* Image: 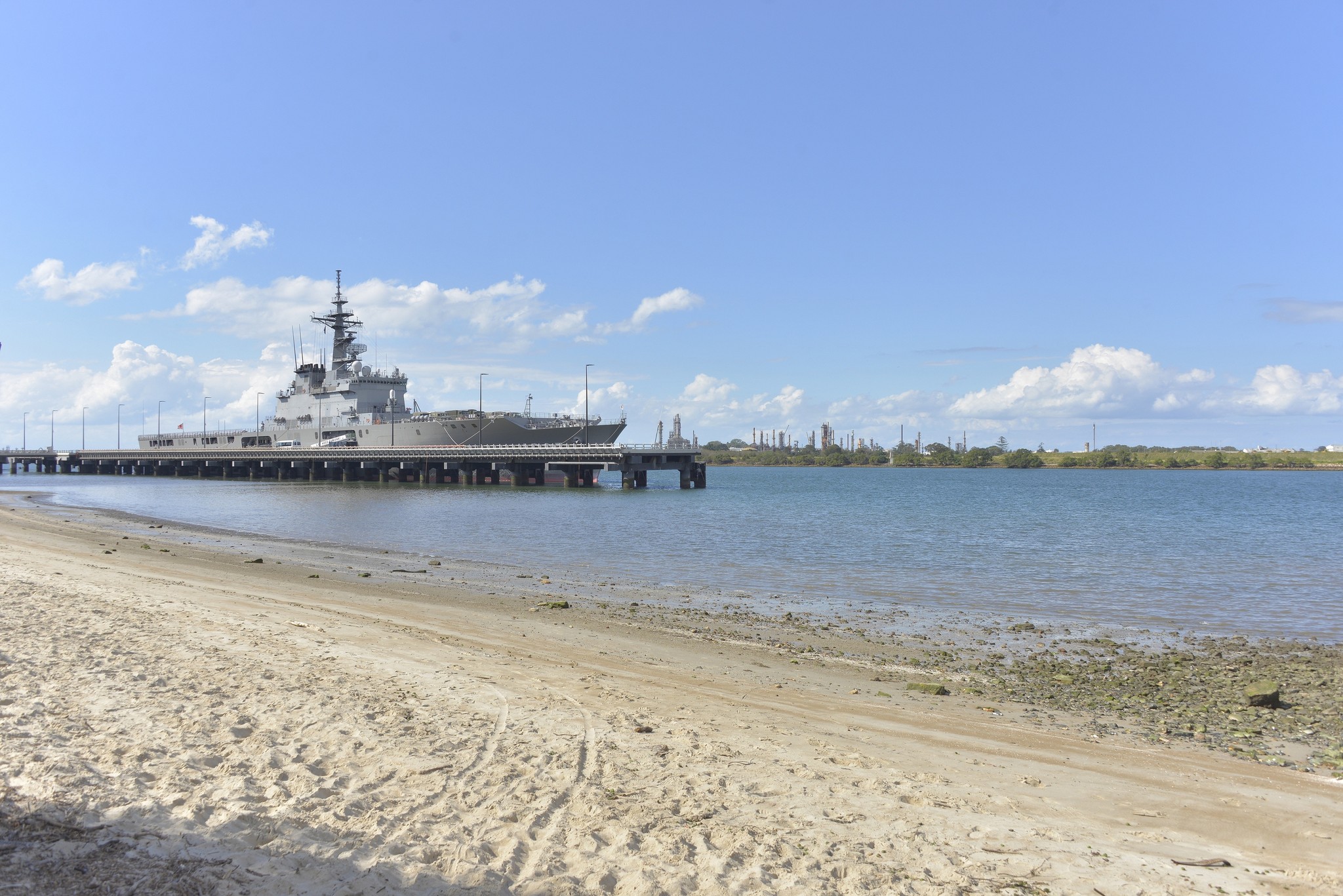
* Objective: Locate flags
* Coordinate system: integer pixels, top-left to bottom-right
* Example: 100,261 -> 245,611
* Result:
324,326 -> 326,334
178,423 -> 183,429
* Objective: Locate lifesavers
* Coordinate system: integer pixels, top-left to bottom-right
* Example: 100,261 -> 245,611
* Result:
476,414 -> 480,418
366,419 -> 369,422
621,419 -> 625,423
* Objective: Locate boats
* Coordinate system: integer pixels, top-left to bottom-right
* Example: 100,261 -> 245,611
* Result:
728,446 -> 743,452
138,268 -> 629,470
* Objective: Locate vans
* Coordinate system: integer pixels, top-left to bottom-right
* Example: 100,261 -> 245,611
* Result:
274,440 -> 301,448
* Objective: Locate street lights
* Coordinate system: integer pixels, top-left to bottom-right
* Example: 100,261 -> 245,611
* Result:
82,407 -> 89,450
391,381 -> 399,449
52,410 -> 58,446
157,401 -> 166,449
585,364 -> 595,448
1093,424 -> 1096,450
256,392 -> 264,448
118,404 -> 125,450
479,373 -> 489,448
204,396 -> 212,449
320,385 -> 328,447
24,412 -> 30,450
